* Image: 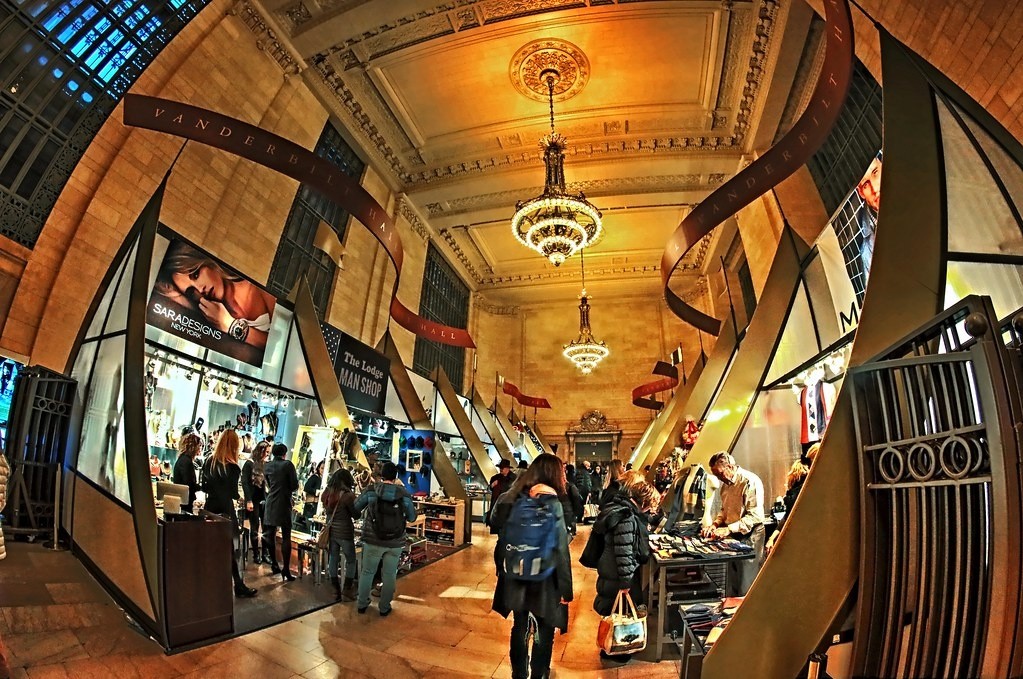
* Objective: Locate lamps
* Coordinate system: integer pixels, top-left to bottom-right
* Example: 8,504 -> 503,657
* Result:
562,248 -> 609,378
148,348 -> 290,407
510,78 -> 603,269
354,413 -> 399,433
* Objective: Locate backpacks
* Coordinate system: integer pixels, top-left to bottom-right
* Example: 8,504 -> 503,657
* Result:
368,490 -> 406,540
505,492 -> 560,581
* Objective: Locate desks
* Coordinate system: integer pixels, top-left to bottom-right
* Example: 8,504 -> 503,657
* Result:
649,537 -> 755,663
679,597 -> 739,679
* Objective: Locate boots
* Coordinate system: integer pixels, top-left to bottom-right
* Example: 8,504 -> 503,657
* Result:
261,537 -> 271,564
250,533 -> 263,563
233,579 -> 258,597
333,584 -> 341,600
342,577 -> 356,600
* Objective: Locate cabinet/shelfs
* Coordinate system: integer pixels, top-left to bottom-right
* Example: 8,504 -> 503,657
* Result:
406,498 -> 464,547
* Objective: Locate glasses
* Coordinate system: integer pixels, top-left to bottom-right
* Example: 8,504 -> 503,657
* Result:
621,465 -> 625,468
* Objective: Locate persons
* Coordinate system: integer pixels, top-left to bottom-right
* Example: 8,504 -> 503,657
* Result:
241,441 -> 273,565
783,442 -> 821,520
354,467 -> 375,495
174,433 -> 202,515
263,442 -> 299,582
488,460 -> 652,534
490,453 -> 573,679
673,462 -> 720,522
0,433 -> 11,679
202,428 -> 259,597
153,239 -> 276,348
354,461 -> 418,616
593,481 -> 661,664
303,461 -> 325,530
856,150 -> 881,288
321,469 -> 362,602
702,451 -> 766,597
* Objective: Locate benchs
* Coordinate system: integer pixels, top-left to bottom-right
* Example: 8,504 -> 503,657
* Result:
244,520 -> 316,579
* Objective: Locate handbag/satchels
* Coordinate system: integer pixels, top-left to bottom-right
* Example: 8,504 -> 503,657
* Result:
596,590 -> 647,655
582,493 -> 600,521
579,529 -> 604,568
316,526 -> 329,549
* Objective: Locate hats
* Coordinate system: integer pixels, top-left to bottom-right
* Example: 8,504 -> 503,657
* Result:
396,436 -> 436,487
381,462 -> 396,479
496,459 -> 513,469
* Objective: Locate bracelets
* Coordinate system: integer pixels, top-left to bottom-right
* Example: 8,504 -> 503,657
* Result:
228,318 -> 249,343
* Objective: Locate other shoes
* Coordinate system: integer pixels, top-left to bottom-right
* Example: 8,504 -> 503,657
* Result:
380,607 -> 391,617
357,606 -> 367,614
600,649 -> 631,663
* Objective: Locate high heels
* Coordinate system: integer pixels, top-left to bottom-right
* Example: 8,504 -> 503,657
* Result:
282,569 -> 295,581
271,562 -> 282,574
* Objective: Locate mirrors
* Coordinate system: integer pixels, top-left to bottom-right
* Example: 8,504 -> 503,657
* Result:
278,425 -> 333,540
